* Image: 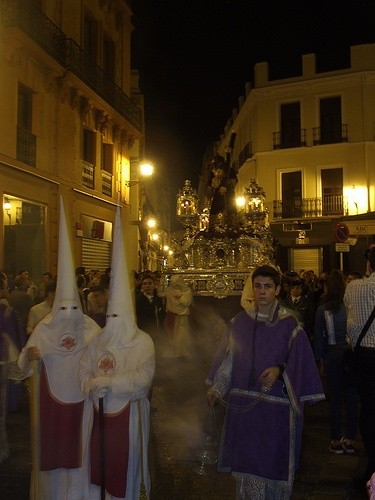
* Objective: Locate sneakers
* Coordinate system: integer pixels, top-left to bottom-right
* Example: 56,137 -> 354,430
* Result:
329,437 -> 354,454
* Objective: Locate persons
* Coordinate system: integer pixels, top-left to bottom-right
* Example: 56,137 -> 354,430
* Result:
18,195 -> 102,500
78,207 -> 156,500
0,269 -> 198,464
205,264 -> 327,500
276,246 -> 375,500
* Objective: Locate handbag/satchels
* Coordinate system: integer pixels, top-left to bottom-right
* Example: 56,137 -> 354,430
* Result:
340,349 -> 360,387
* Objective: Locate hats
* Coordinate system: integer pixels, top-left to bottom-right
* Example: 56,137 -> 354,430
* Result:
288,280 -> 305,287
209,155 -> 226,168
